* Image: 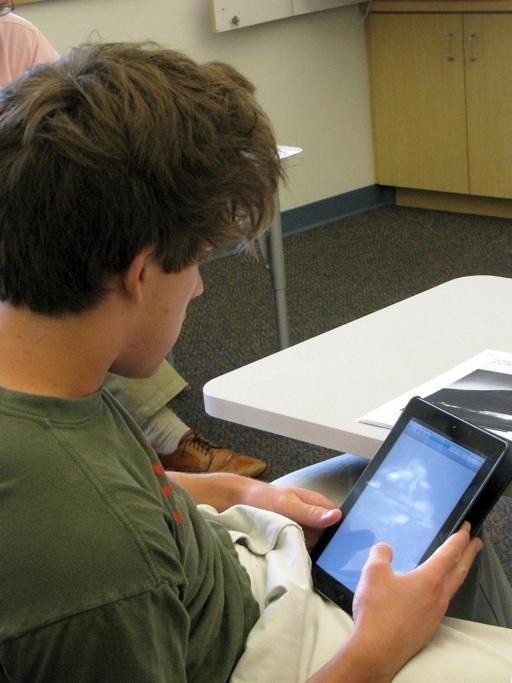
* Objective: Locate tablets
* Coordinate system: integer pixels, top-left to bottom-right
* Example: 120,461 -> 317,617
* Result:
311,396 -> 509,620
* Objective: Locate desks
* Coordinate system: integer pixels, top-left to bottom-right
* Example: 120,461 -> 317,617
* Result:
202,274 -> 512,461
266,143 -> 306,349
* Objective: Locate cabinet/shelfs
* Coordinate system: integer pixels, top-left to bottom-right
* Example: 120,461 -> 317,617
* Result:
364,12 -> 512,221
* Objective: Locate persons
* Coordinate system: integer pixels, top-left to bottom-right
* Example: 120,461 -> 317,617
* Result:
0,37 -> 512,683
0,1 -> 268,479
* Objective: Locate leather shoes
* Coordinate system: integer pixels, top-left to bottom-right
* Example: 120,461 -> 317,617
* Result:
158,429 -> 267,479
